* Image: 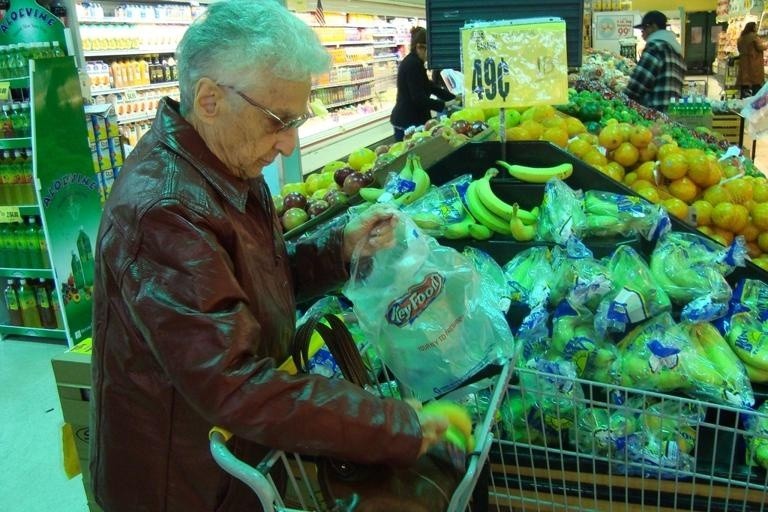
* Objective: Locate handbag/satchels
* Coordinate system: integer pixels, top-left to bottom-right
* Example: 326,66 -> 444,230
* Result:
292,311 -> 490,511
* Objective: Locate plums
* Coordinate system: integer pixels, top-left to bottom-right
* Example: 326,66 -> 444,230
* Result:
275,118 -> 488,234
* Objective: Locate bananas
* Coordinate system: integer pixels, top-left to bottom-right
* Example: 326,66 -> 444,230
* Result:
346,153 -> 768,466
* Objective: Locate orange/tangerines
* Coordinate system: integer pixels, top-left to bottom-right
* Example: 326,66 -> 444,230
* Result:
558,81 -> 766,177
488,104 -> 767,271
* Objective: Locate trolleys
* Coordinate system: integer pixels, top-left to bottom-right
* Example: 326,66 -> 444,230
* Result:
207,292 -> 767,511
715,55 -> 741,100
682,65 -> 710,98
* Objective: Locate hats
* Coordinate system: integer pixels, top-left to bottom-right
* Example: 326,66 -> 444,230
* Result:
632,11 -> 668,29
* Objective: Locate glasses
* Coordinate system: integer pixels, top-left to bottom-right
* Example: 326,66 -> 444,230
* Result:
214,82 -> 313,133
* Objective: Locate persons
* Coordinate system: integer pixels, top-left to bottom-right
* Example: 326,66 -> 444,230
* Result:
89,0 -> 448,512
737,21 -> 768,100
626,11 -> 686,114
390,28 -> 457,142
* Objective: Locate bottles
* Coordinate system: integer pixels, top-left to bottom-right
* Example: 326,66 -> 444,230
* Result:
77,3 -> 406,151
1,42 -> 67,331
70,250 -> 85,289
621,45 -> 638,62
666,91 -> 714,131
77,223 -> 97,287
